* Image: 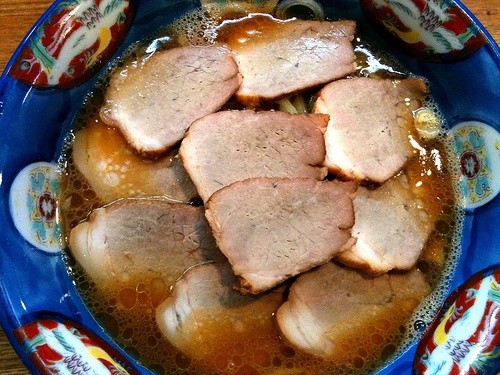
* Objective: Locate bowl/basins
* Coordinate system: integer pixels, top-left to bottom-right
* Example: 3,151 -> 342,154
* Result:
0,0 -> 500,375
